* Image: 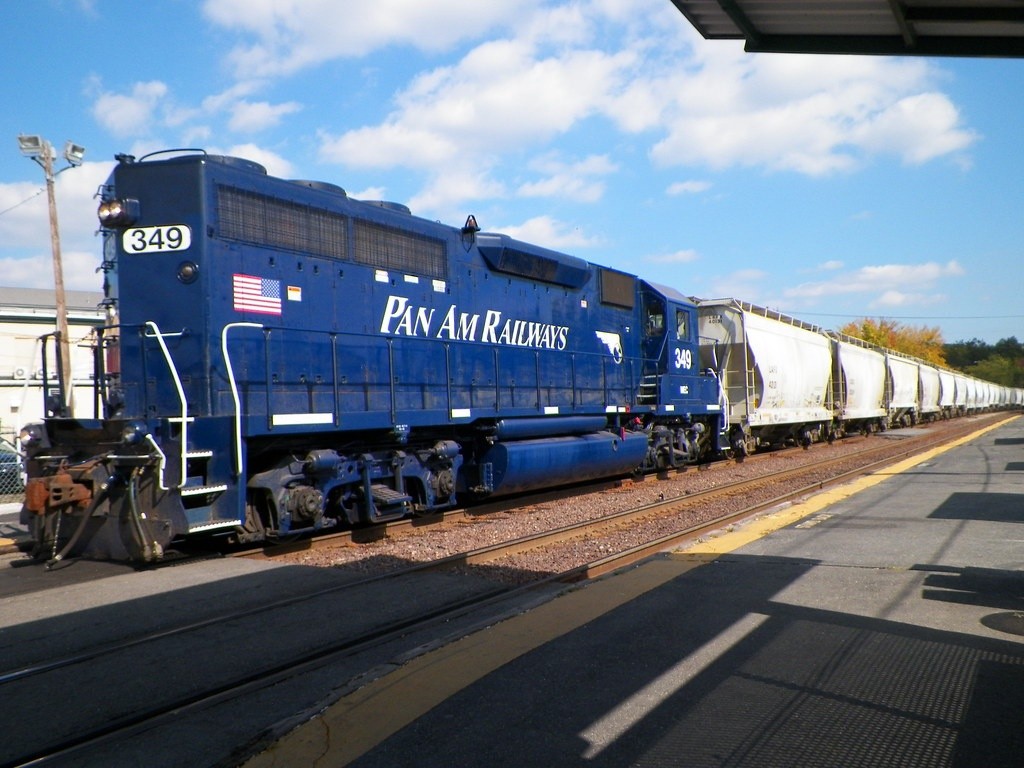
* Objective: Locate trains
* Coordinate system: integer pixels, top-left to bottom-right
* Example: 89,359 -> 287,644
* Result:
15,142 -> 1024,572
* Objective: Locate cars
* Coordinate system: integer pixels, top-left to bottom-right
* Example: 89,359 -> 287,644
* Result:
0,438 -> 27,495
